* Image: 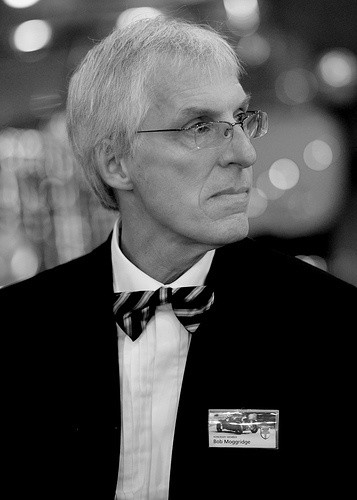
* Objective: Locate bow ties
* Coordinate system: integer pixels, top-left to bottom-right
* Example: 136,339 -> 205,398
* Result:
109,288 -> 214,342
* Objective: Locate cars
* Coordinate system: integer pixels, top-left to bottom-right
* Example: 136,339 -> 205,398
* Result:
216,416 -> 258,434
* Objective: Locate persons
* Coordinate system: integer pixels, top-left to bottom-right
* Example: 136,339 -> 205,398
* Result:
0,18 -> 357,500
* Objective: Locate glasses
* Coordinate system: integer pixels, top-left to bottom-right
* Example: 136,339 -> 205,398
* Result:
134,110 -> 269,147
